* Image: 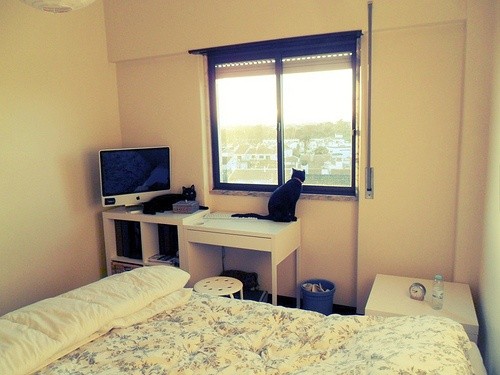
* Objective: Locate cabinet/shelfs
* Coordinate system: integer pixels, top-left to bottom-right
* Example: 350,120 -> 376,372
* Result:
101,206 -> 224,288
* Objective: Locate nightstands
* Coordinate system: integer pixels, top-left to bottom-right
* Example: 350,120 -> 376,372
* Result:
364,273 -> 479,345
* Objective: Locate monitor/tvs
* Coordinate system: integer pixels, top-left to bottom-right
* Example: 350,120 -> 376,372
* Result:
98,146 -> 172,214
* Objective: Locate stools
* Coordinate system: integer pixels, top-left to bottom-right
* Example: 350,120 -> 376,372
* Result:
194,276 -> 243,301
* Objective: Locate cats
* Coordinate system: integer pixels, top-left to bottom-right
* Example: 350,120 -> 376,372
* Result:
232,168 -> 306,222
143,184 -> 209,216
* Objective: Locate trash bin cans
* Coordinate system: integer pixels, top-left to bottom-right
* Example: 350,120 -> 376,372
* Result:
299,277 -> 337,316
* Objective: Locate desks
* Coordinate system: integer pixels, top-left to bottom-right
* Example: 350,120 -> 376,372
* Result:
186,212 -> 302,310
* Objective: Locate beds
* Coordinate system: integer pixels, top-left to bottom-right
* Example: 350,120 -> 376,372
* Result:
0,265 -> 491,375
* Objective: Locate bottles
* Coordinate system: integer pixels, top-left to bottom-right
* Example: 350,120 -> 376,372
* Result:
430,272 -> 444,310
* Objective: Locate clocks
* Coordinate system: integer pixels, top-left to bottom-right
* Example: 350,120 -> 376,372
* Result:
409,283 -> 426,301
